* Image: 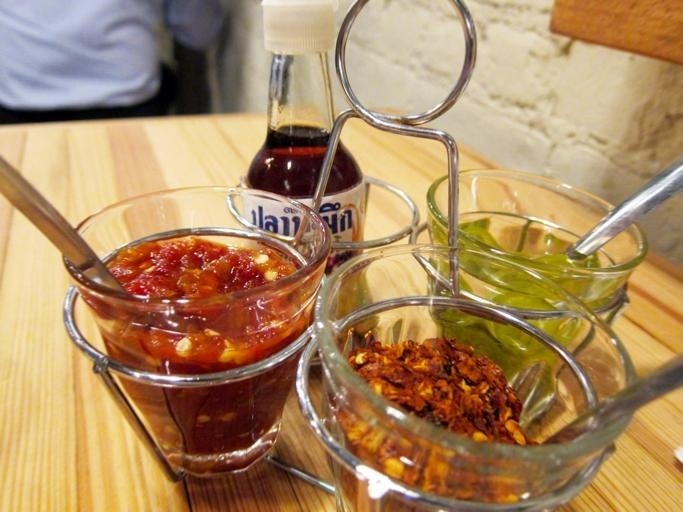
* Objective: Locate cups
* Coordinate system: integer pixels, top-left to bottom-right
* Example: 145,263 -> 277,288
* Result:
63,187 -> 327,480
427,166 -> 647,427
314,243 -> 640,512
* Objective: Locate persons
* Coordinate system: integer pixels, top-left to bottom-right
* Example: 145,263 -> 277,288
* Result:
0,1 -> 225,125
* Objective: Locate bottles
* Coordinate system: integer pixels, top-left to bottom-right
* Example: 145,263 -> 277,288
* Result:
244,0 -> 371,382
